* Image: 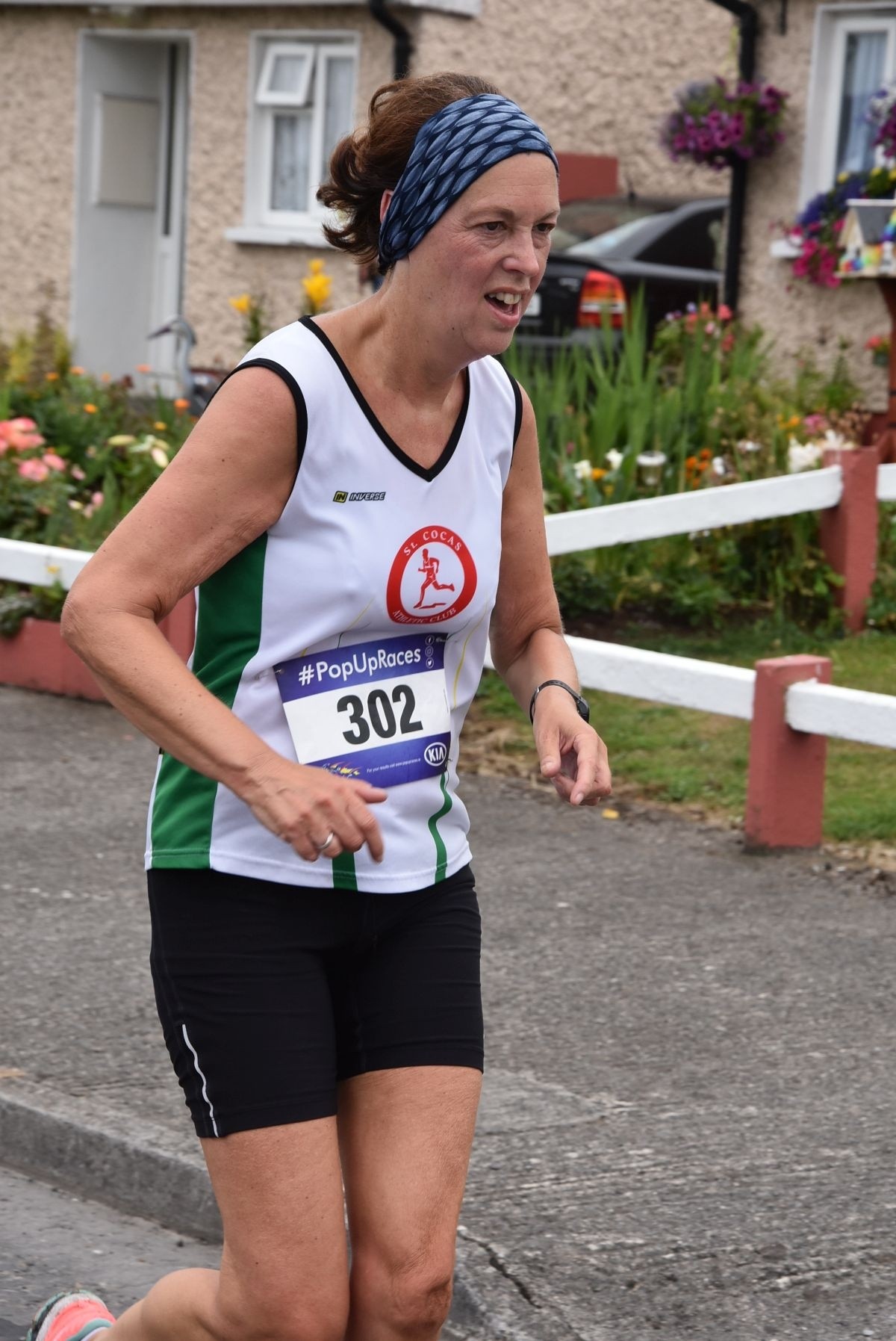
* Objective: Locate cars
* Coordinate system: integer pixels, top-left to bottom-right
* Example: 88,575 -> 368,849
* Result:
506,187 -> 727,369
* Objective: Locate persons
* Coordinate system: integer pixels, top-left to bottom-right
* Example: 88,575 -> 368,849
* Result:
21,70 -> 612,1341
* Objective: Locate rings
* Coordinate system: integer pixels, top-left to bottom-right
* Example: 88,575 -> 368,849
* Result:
315,831 -> 334,849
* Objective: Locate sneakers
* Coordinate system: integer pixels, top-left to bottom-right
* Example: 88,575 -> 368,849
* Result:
28,1289 -> 119,1341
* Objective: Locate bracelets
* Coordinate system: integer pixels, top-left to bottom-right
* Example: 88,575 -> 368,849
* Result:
529,680 -> 590,724
